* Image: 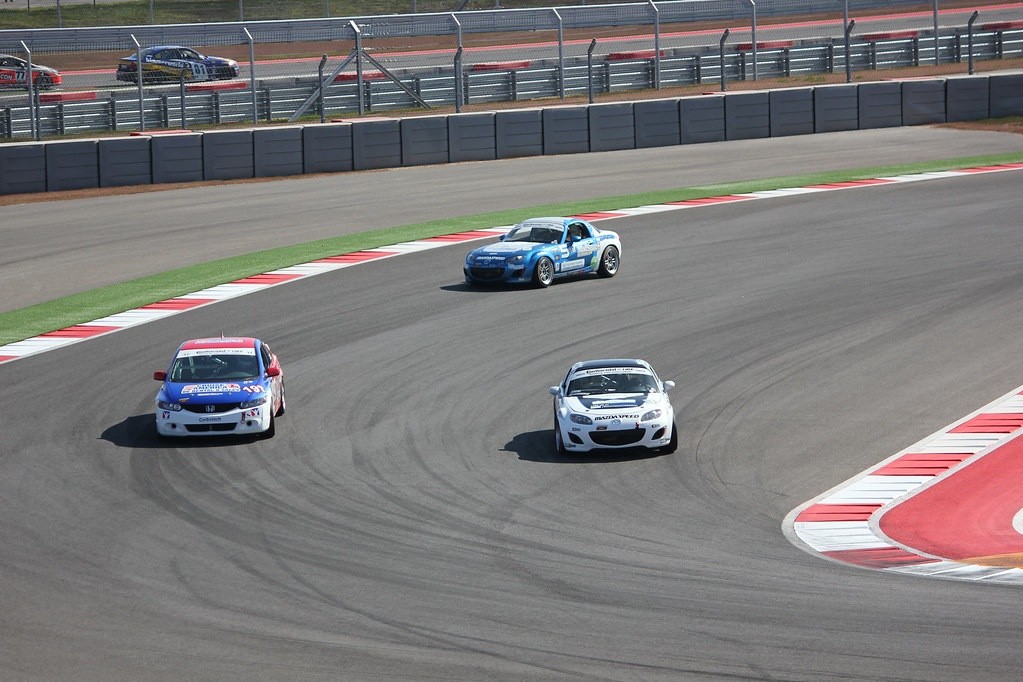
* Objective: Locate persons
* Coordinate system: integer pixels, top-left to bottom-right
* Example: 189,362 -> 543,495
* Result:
215,358 -> 241,376
615,378 -> 651,391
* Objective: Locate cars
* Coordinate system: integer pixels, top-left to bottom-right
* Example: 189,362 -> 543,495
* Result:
154,332 -> 287,440
0,51 -> 63,90
547,354 -> 678,456
463,215 -> 624,286
117,45 -> 242,84
